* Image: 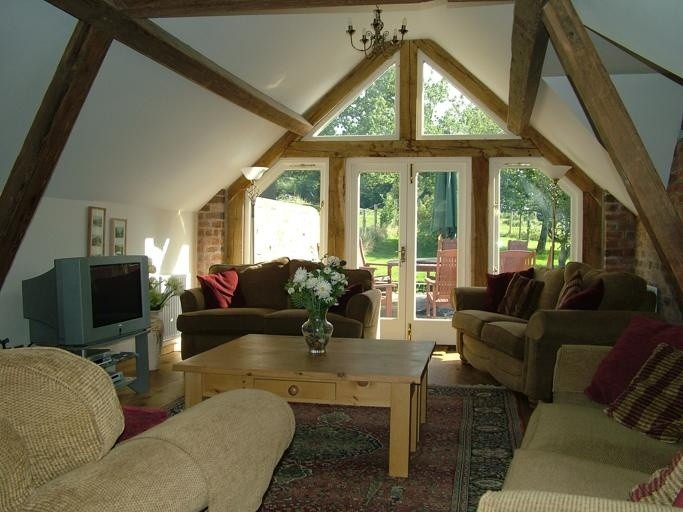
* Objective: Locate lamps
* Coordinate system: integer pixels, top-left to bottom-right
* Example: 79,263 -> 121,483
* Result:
241,166 -> 269,264
344,2 -> 409,59
540,164 -> 572,267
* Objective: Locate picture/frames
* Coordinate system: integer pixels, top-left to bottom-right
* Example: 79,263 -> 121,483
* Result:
85,206 -> 128,257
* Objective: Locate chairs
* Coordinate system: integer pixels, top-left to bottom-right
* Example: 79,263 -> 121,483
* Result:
0,346 -> 296,509
424,232 -> 535,318
356,237 -> 397,316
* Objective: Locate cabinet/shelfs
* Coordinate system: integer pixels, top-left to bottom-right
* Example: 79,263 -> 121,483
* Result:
70,329 -> 150,396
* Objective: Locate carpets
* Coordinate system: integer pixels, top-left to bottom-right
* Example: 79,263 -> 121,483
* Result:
157,383 -> 529,512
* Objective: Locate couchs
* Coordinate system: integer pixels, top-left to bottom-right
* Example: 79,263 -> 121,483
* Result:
174,254 -> 381,387
452,260 -> 664,409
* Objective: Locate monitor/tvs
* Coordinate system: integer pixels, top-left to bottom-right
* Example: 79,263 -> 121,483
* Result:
21,255 -> 150,345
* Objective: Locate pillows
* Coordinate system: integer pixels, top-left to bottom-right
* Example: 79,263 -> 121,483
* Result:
555,269 -> 605,310
585,316 -> 681,441
482,265 -> 544,318
194,268 -> 245,311
626,447 -> 683,510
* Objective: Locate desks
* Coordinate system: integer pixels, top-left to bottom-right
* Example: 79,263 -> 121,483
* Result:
387,256 -> 441,284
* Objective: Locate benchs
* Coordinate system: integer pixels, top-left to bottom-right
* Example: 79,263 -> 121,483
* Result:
480,342 -> 682,510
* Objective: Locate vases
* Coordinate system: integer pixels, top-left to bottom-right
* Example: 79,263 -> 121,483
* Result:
300,310 -> 335,355
147,311 -> 165,371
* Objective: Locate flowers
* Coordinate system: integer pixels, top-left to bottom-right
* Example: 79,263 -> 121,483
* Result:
147,263 -> 183,311
282,255 -> 350,344
